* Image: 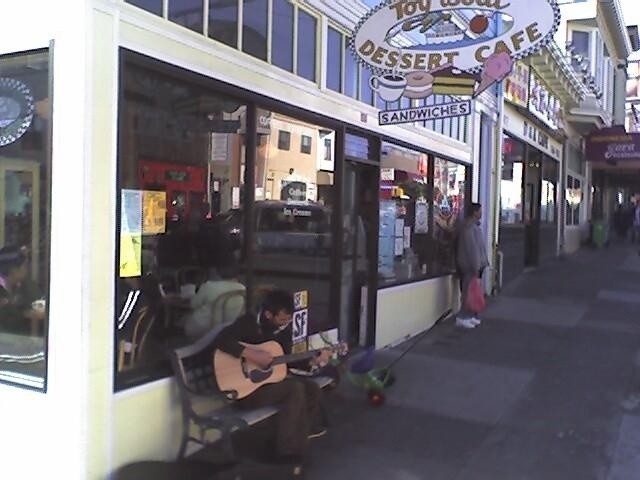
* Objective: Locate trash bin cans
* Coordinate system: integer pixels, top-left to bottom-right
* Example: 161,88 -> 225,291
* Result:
591,222 -> 611,248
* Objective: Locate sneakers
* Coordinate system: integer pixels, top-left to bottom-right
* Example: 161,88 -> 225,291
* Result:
455,315 -> 481,330
306,429 -> 328,440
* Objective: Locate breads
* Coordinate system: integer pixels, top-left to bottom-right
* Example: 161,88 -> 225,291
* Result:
402,72 -> 434,99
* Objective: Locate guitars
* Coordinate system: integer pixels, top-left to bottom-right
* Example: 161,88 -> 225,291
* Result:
214,341 -> 348,402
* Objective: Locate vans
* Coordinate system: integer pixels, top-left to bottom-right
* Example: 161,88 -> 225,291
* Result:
252,199 -> 334,258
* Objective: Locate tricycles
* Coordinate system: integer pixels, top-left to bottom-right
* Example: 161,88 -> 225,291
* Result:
313,308 -> 455,404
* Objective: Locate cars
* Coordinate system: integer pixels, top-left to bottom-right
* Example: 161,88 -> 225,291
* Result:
217,207 -> 241,250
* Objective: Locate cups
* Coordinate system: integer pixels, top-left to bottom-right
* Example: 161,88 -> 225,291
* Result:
367,73 -> 407,103
32,300 -> 46,312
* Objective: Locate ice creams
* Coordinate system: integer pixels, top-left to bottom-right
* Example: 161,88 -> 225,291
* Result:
472,52 -> 513,98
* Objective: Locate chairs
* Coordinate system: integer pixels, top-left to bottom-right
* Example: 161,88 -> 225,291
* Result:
1,260 -> 254,370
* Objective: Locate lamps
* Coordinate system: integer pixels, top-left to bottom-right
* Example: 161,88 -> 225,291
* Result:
558,38 -> 604,101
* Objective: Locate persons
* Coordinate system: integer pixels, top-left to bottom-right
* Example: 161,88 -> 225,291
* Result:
0,246 -> 247,366
454,203 -> 490,330
629,192 -> 640,245
205,291 -> 327,468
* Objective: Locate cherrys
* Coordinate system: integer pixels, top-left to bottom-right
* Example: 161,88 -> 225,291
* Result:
470,15 -> 487,33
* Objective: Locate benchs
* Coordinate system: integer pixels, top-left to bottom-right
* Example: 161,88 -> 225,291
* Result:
170,319 -> 335,464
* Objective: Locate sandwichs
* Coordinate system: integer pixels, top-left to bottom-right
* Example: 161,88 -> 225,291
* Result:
432,65 -> 475,95
